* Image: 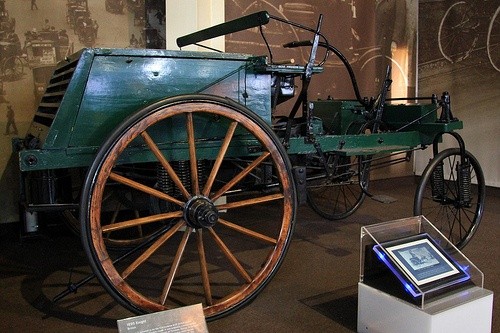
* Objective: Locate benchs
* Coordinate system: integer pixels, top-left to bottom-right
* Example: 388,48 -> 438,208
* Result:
176,11 -> 329,137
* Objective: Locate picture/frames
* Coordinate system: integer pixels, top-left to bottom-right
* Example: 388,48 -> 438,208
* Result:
374,232 -> 470,297
